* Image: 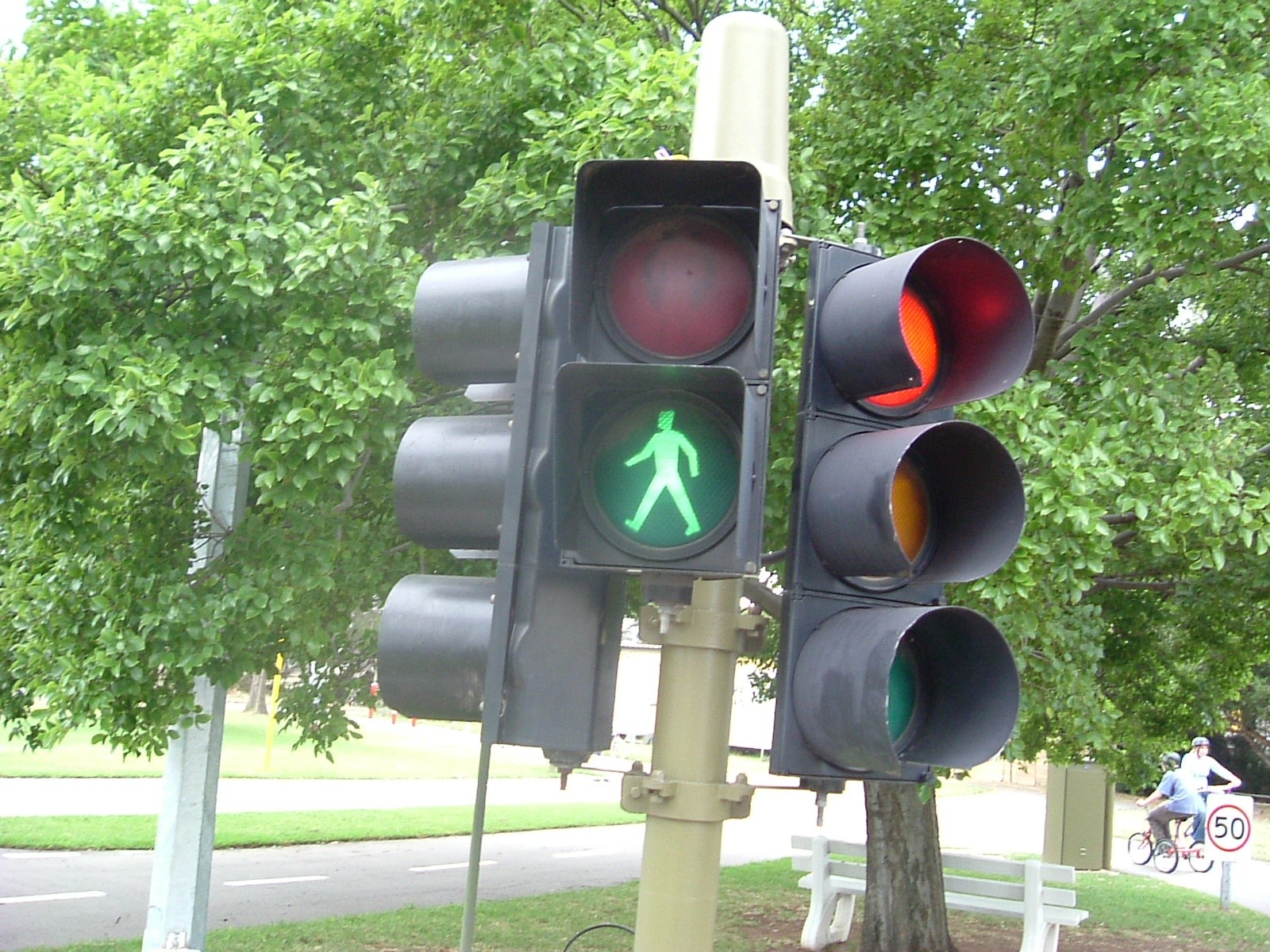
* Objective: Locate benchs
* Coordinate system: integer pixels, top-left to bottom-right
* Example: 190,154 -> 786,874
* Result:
792,836 -> 1089,952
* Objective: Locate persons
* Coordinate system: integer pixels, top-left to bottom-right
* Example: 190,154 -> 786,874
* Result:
1181,737 -> 1241,851
1135,752 -> 1198,856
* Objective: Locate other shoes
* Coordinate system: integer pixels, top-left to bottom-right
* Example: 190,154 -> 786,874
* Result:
1154,844 -> 1168,856
1164,848 -> 1174,858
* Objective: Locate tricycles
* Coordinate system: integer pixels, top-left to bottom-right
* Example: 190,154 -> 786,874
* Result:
1128,805 -> 1214,874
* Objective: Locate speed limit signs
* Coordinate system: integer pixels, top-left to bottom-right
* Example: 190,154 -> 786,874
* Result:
1206,802 -> 1251,852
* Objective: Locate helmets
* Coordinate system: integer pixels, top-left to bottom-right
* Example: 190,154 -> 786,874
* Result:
1159,752 -> 1181,768
1191,736 -> 1209,747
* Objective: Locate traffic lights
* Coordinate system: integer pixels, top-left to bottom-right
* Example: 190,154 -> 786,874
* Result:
769,233 -> 1036,783
547,158 -> 784,578
377,221 -> 626,790
